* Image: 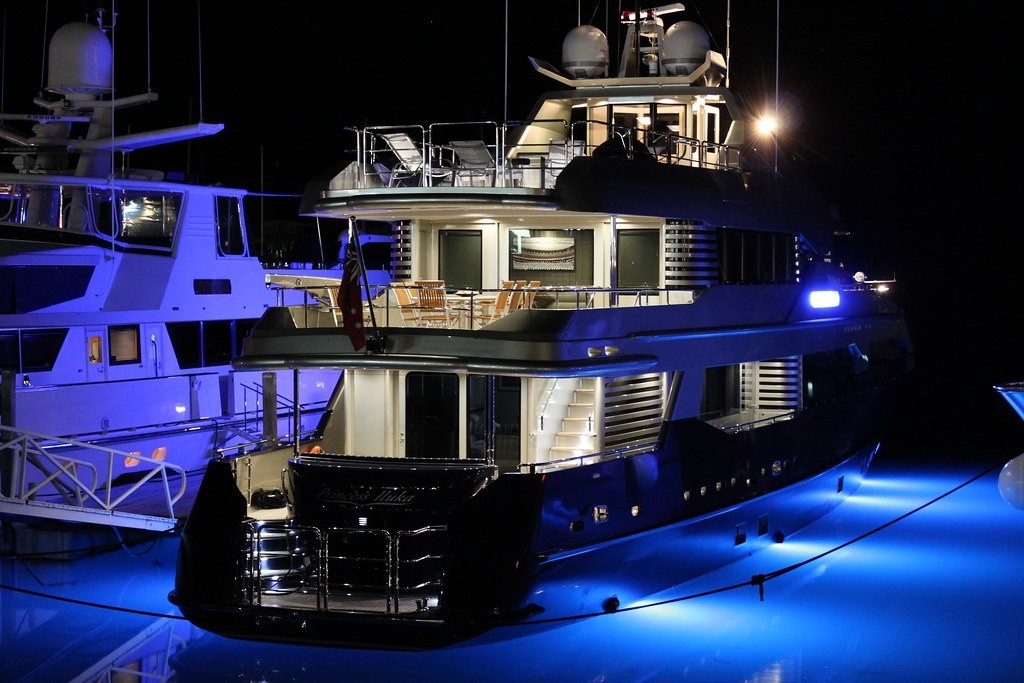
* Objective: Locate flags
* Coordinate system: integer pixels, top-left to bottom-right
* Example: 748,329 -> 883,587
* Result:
336,218 -> 367,352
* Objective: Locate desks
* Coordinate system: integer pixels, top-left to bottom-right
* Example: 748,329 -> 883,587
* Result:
410,295 -> 497,330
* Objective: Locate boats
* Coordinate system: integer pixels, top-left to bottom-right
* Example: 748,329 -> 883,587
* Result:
0,0 -> 394,565
165,0 -> 915,653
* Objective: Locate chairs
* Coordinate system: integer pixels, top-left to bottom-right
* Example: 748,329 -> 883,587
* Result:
379,134 -> 586,192
390,279 -> 541,331
